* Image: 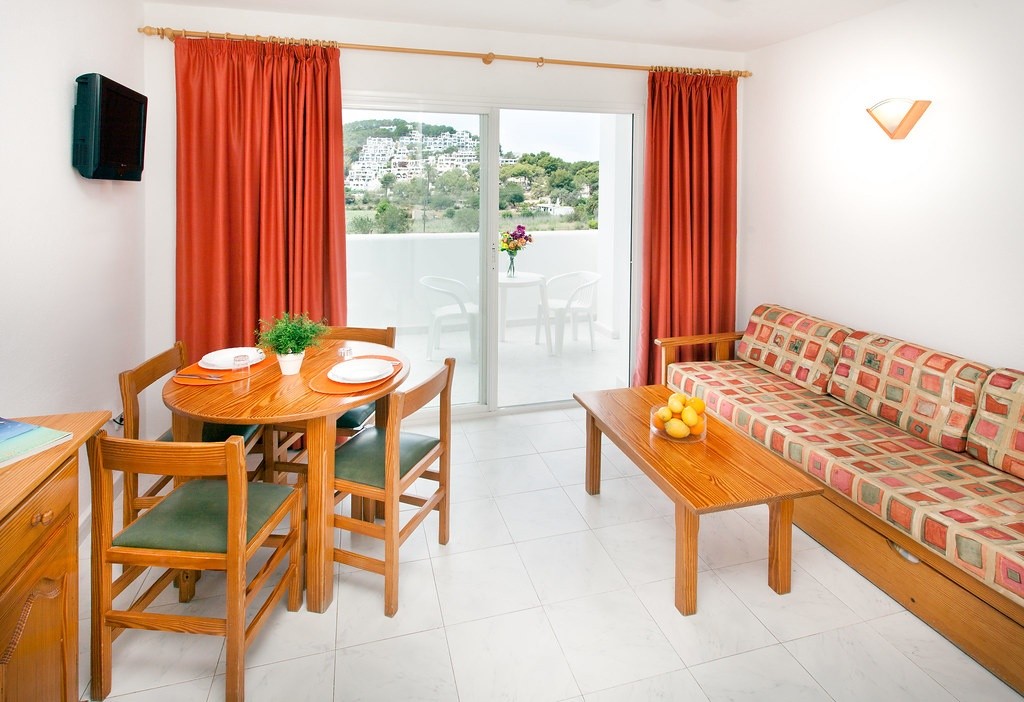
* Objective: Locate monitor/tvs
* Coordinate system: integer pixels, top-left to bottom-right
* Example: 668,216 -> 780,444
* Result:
72,72 -> 148,181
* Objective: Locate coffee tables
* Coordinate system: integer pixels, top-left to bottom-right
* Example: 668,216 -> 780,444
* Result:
573,384 -> 824,615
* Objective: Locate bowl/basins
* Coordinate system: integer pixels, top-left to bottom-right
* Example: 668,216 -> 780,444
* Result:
649,403 -> 708,443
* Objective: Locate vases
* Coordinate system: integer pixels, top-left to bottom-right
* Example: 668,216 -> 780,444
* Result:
505,252 -> 520,277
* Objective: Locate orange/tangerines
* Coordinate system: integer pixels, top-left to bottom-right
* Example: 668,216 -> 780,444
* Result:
652,393 -> 705,435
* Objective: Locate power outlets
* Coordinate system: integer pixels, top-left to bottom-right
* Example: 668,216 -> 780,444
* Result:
113,410 -> 123,430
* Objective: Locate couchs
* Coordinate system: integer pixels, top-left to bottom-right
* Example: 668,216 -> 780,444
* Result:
654,303 -> 1023,701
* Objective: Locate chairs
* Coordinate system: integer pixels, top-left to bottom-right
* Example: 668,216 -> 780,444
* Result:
301,355 -> 455,616
419,275 -> 479,368
272,325 -> 401,524
536,271 -> 602,355
117,337 -> 276,584
78,431 -> 305,702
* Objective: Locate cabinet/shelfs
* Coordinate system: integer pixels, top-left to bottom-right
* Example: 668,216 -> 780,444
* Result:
0,408 -> 117,702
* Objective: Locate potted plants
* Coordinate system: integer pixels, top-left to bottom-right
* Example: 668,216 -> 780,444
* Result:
251,311 -> 328,379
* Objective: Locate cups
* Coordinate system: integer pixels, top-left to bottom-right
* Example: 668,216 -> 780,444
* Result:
231,355 -> 250,394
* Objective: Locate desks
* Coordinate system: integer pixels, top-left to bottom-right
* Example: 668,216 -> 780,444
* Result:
477,270 -> 550,347
162,341 -> 407,613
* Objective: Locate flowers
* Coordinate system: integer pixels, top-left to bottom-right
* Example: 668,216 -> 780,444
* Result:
498,224 -> 535,254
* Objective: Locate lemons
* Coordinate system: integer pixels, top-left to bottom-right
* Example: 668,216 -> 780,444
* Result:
658,399 -> 698,439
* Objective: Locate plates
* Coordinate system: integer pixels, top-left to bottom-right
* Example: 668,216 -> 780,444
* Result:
198,346 -> 266,370
327,359 -> 394,384
